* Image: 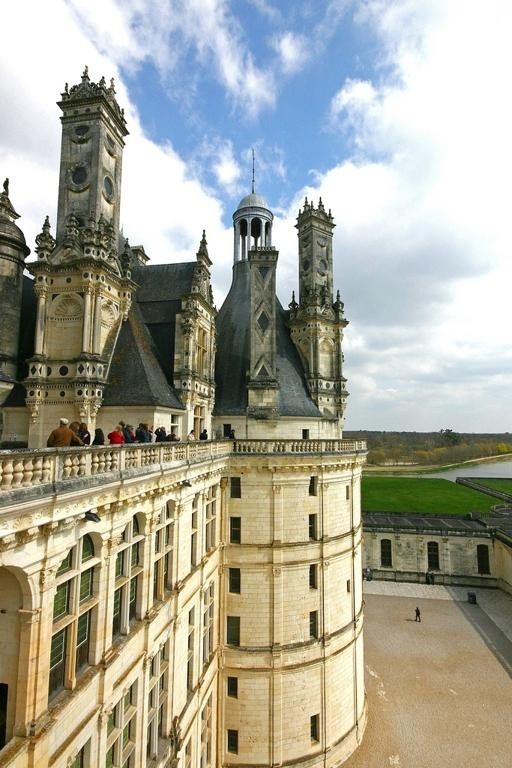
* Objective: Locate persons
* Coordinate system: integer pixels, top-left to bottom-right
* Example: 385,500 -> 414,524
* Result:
365,566 -> 372,581
431,573 -> 435,585
46,418 -> 237,447
426,572 -> 430,585
414,607 -> 421,622
